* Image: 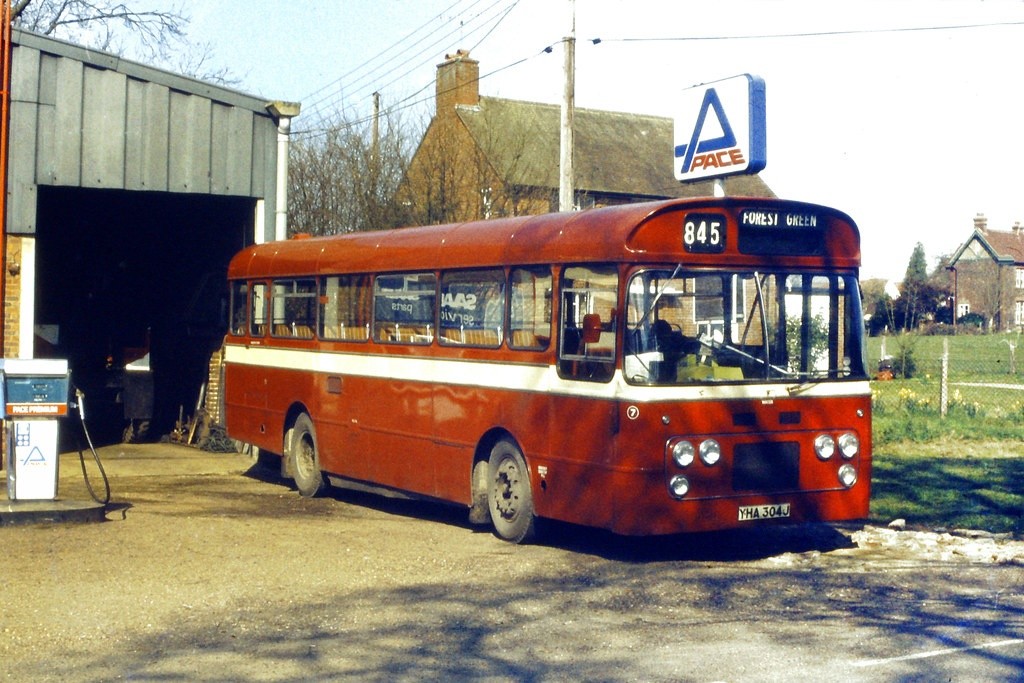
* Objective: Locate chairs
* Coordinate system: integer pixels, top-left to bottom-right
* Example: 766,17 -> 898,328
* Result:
237,321 -> 542,348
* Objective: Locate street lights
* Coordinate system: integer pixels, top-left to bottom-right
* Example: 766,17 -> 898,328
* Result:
944,266 -> 957,334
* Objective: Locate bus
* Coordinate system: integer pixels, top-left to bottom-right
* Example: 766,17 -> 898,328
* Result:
223,194 -> 873,550
